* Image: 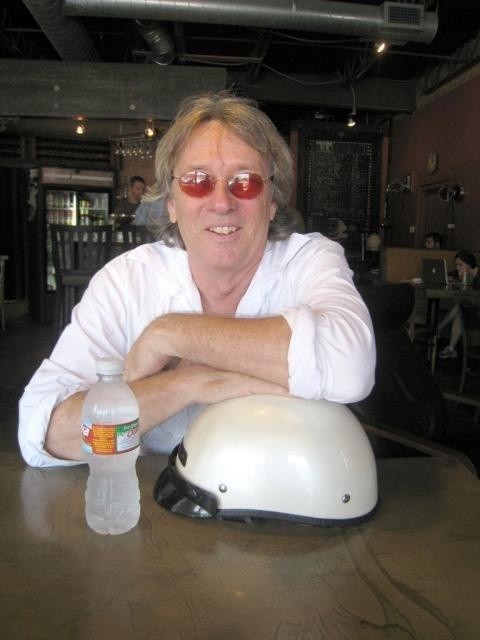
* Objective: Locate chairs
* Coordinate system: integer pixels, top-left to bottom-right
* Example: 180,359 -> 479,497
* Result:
402,283 -> 480,406
46,223 -> 157,341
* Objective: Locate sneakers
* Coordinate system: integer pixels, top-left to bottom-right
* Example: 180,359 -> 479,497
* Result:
437,347 -> 458,358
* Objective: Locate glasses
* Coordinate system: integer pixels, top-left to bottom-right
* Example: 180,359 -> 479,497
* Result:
170,167 -> 275,201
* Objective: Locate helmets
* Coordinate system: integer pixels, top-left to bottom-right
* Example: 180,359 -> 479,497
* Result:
153,393 -> 380,529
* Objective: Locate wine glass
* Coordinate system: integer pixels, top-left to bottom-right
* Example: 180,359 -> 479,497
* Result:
115,143 -> 156,162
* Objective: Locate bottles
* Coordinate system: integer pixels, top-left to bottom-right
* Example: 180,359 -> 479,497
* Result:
80,357 -> 141,537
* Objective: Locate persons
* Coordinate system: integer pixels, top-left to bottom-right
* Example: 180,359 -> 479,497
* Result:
15,98 -> 377,469
115,176 -> 151,230
436,250 -> 480,359
424,232 -> 443,249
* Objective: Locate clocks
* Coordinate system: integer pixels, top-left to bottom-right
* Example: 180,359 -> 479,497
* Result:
426,150 -> 439,174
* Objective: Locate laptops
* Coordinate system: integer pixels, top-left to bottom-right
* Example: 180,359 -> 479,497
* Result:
422,256 -> 456,286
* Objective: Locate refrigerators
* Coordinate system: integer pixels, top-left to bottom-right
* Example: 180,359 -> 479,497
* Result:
40,167 -> 116,294
